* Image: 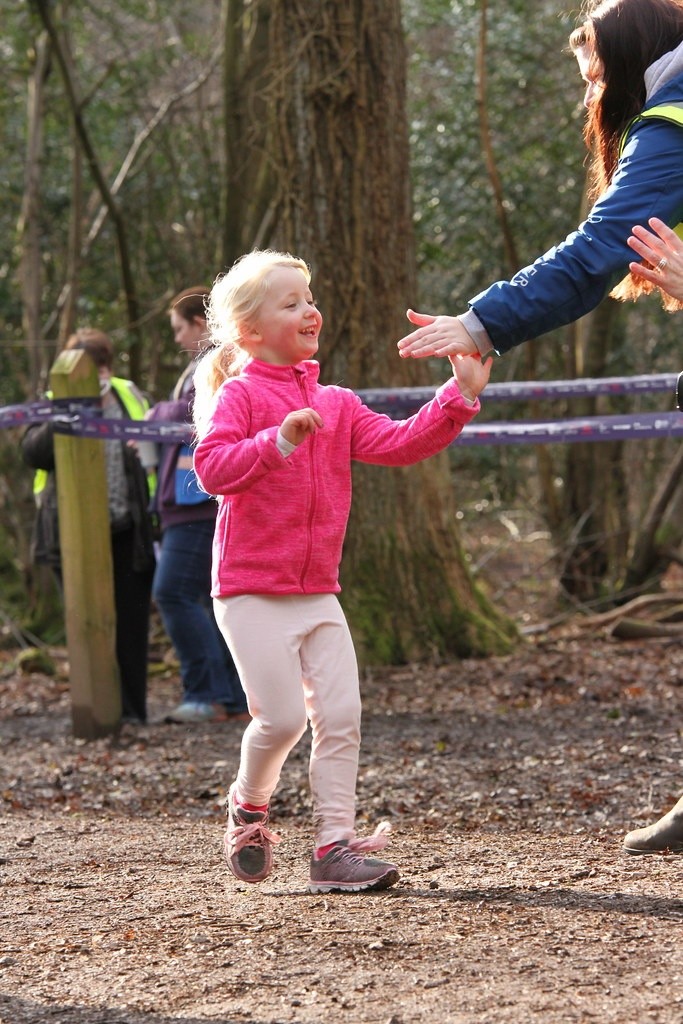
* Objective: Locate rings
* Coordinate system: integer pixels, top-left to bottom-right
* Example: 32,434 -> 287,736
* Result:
656,259 -> 667,272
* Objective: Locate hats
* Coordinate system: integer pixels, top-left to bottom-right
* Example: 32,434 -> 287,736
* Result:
68,324 -> 112,370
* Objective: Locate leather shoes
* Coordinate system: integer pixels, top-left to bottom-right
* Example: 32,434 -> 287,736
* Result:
622,793 -> 682,855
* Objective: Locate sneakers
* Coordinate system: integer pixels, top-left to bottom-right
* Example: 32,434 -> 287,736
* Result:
164,696 -> 226,728
309,820 -> 400,894
227,782 -> 282,881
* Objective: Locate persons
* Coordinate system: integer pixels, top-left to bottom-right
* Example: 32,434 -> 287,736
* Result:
397,0 -> 683,857
18,327 -> 156,730
193,248 -> 493,894
128,285 -> 252,727
